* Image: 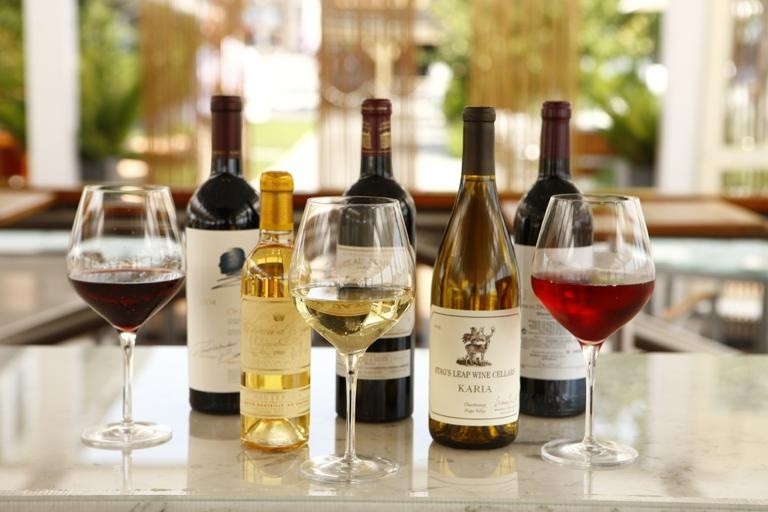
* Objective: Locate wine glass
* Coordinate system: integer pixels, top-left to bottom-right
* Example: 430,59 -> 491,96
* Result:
64,184 -> 186,451
528,191 -> 658,470
287,194 -> 418,484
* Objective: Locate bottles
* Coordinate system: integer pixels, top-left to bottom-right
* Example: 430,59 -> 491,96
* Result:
428,105 -> 520,448
334,95 -> 414,422
514,101 -> 594,417
186,94 -> 259,415
241,172 -> 312,453
